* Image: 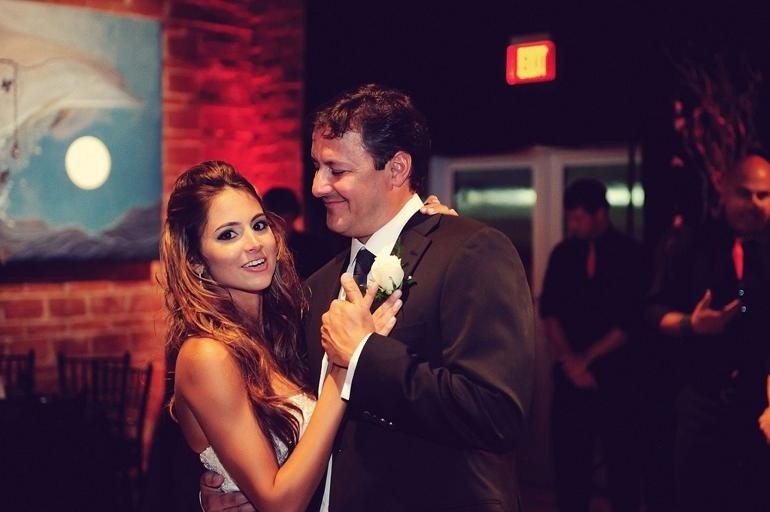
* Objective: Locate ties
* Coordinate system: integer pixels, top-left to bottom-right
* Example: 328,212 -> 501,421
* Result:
345,249 -> 376,303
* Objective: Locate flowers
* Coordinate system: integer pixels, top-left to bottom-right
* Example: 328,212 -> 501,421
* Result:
371,243 -> 417,300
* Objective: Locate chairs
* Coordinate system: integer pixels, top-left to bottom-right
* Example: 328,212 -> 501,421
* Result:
0,349 -> 152,512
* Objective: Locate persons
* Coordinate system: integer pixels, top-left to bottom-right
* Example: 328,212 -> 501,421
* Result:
646,152 -> 770,512
538,177 -> 636,512
261,187 -> 299,241
162,160 -> 457,512
310,84 -> 539,512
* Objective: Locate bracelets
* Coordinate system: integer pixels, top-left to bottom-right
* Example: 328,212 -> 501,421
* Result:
333,363 -> 347,369
